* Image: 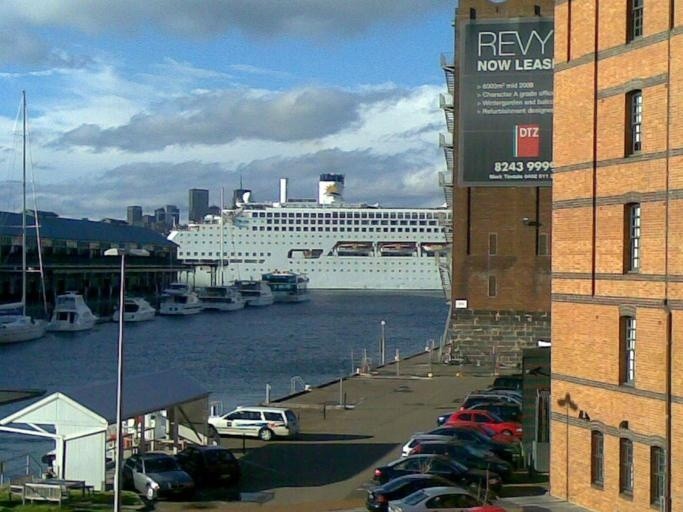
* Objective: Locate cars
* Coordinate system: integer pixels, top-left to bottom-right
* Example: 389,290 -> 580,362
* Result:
364,374 -> 524,512
121,439 -> 243,500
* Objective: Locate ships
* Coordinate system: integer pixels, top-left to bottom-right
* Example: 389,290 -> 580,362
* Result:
166,206 -> 452,296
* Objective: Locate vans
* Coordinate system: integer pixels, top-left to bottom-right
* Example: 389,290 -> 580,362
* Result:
208,405 -> 299,440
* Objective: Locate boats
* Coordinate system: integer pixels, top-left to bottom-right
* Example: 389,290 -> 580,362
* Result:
49,272 -> 309,331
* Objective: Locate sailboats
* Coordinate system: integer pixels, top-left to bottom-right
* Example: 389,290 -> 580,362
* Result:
0,85 -> 47,343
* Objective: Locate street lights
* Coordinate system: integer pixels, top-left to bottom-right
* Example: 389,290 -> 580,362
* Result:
99,244 -> 149,511
379,320 -> 387,365
